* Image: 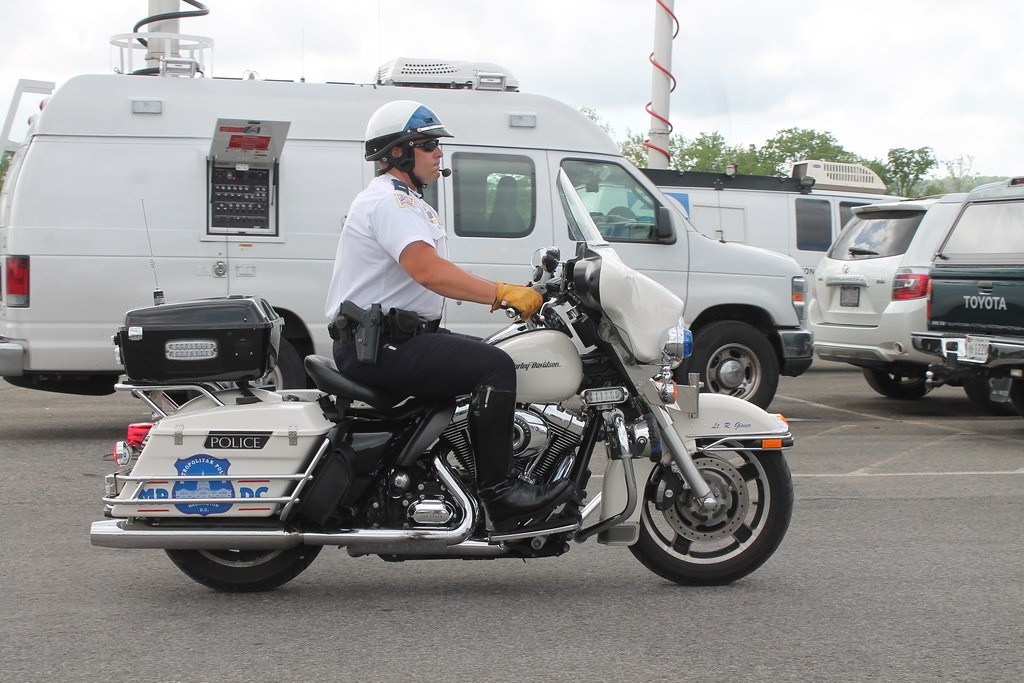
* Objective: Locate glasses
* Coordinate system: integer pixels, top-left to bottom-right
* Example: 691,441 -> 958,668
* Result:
414,140 -> 439,152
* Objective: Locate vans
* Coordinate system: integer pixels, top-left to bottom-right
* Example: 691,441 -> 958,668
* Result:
0,0 -> 814,411
574,158 -> 888,279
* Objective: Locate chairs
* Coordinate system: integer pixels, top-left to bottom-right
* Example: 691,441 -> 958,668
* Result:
488,176 -> 526,232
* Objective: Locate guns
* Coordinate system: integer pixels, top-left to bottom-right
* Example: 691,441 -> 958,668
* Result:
340,298 -> 384,363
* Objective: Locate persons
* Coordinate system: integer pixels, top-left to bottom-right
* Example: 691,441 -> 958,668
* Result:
325,100 -> 576,532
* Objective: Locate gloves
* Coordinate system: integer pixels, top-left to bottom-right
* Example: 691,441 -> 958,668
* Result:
490,281 -> 542,321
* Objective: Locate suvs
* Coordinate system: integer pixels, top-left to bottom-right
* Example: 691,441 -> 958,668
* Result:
911,174 -> 1024,418
807,193 -> 969,400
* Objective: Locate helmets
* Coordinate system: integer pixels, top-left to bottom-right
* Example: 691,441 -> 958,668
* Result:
365,100 -> 454,160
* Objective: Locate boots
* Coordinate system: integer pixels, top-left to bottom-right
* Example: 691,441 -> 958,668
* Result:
467,385 -> 573,531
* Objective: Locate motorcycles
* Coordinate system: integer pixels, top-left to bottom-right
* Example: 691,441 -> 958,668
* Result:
89,164 -> 794,594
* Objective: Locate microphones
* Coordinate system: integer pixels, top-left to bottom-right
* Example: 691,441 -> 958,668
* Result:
437,168 -> 452,177
